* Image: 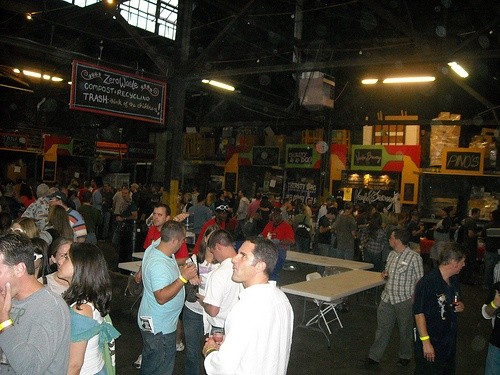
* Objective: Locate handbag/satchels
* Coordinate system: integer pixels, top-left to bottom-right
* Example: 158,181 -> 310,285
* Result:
184,253 -> 200,302
296,216 -> 311,238
111,218 -> 126,248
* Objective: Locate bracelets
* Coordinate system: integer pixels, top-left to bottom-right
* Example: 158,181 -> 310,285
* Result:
179,275 -> 188,284
0,319 -> 13,330
419,336 -> 430,340
329,226 -> 332,229
206,349 -> 216,356
491,301 -> 498,309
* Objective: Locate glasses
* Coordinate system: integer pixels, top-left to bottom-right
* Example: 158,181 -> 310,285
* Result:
9,227 -> 22,233
217,205 -> 229,211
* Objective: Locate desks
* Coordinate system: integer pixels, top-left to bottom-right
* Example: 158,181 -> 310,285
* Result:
282,250 -> 386,350
118,252 -> 144,310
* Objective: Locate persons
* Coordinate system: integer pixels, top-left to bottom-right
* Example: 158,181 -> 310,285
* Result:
475,281 -> 500,375
134,204 -> 294,375
111,182 -> 166,244
0,179 -> 121,375
411,242 -> 466,375
355,231 -> 424,372
175,182 -> 500,305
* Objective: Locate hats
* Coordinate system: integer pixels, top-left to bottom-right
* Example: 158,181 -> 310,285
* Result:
443,207 -> 450,215
46,192 -> 66,201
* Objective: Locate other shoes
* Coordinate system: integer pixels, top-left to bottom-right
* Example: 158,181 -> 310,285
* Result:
283,266 -> 295,271
397,358 -> 410,366
175,342 -> 185,351
134,355 -> 143,369
359,358 -> 379,366
